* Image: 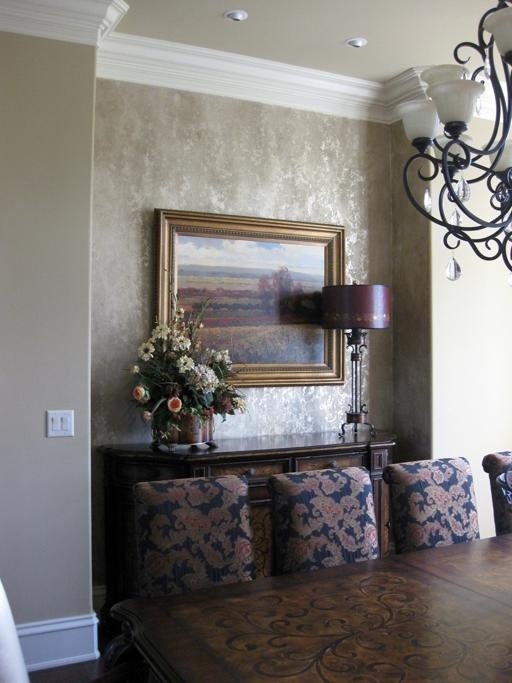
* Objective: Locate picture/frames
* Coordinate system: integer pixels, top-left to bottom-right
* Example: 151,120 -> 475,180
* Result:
151,204 -> 347,386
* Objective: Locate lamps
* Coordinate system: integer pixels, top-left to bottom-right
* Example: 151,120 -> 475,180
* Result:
396,0 -> 512,281
319,281 -> 391,438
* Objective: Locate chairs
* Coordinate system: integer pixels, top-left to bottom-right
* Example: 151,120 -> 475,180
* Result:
269,465 -> 382,576
480,451 -> 511,539
382,456 -> 481,556
129,473 -> 258,600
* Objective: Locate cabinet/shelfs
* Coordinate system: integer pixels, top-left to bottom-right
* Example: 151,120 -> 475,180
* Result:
94,435 -> 399,605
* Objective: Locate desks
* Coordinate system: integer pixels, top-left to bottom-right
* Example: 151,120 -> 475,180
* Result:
103,532 -> 511,683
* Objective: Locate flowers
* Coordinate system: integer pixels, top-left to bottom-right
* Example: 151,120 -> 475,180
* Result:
132,289 -> 247,445
